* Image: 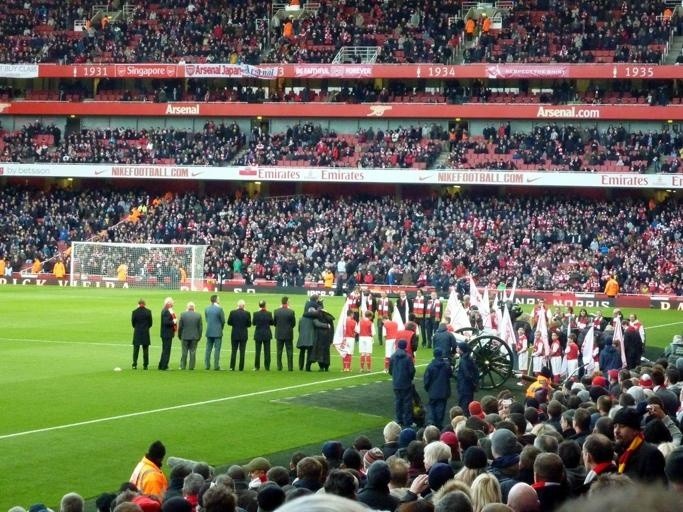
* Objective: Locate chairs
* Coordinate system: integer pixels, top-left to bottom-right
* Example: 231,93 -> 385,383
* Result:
0,1 -> 683,173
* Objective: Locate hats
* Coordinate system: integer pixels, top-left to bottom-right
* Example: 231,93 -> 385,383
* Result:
576,390 -> 591,401
457,342 -> 468,353
434,347 -> 442,358
542,366 -> 551,378
464,396 -> 518,468
323,436 -> 390,484
593,376 -> 606,386
383,422 -> 415,443
440,432 -> 458,451
613,407 -> 640,429
149,441 -> 166,459
608,369 -> 618,379
627,386 -> 644,400
640,374 -> 653,388
572,382 -> 586,392
427,463 -> 455,490
398,340 -> 407,349
243,457 -> 270,472
